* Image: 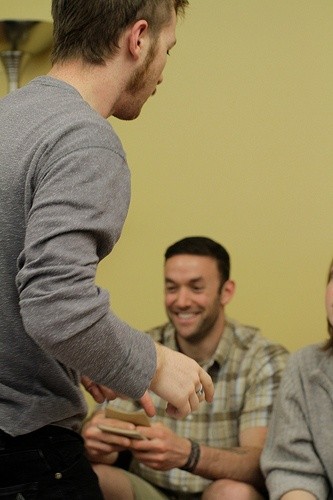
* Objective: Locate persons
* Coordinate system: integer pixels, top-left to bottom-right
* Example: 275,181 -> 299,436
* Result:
258,262 -> 333,500
0,0 -> 215,500
82,235 -> 289,500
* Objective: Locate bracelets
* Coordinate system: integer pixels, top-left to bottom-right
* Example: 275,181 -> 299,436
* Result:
178,437 -> 199,471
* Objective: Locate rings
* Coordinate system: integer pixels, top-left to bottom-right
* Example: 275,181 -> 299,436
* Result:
196,384 -> 203,396
